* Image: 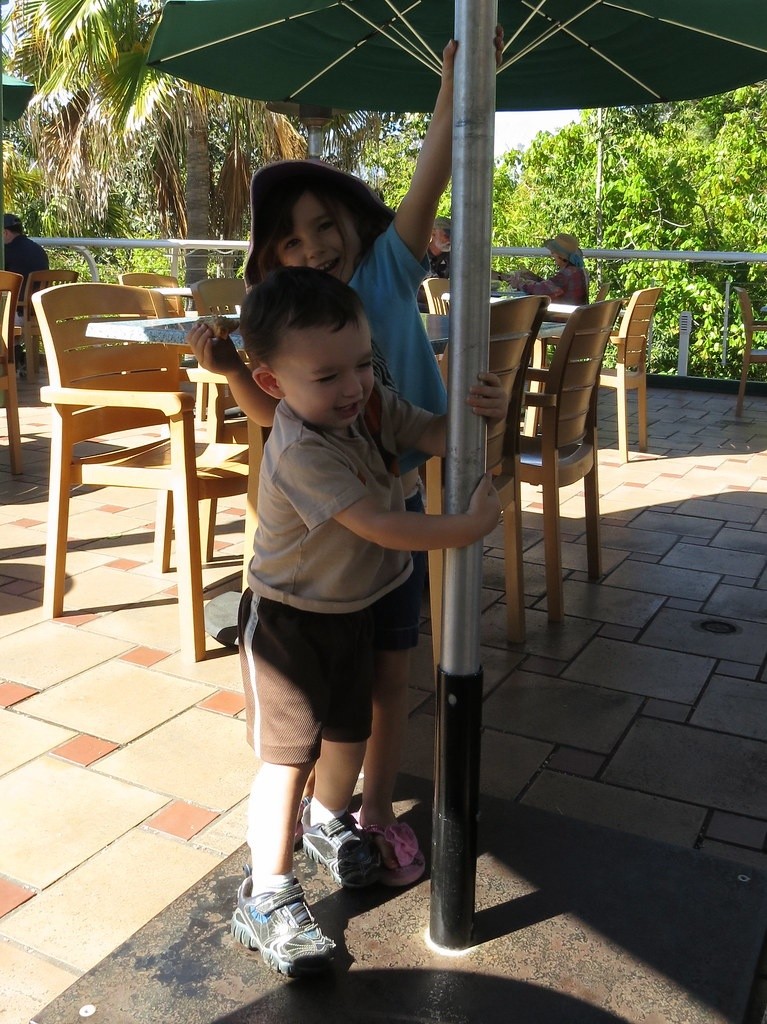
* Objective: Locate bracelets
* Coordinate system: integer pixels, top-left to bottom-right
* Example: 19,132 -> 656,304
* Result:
498,272 -> 502,281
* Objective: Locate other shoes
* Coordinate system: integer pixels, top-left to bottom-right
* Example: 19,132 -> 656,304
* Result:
358,812 -> 427,888
294,796 -> 309,848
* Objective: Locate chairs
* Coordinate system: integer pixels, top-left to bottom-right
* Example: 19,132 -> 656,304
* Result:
1,269 -> 662,664
732,287 -> 766,415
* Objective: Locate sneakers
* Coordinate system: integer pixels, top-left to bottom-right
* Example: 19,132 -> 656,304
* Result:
300,804 -> 381,887
229,866 -> 335,974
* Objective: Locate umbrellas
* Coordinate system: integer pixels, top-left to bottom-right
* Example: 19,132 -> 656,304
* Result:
1,74 -> 36,122
147,0 -> 767,952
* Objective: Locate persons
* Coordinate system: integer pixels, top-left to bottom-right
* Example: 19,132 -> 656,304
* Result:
505,233 -> 590,306
417,216 -> 514,314
222,266 -> 507,978
2,214 -> 50,379
186,26 -> 505,890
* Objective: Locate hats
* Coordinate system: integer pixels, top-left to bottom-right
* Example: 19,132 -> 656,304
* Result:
433,218 -> 452,229
5,214 -> 22,233
544,232 -> 584,266
243,158 -> 396,291
